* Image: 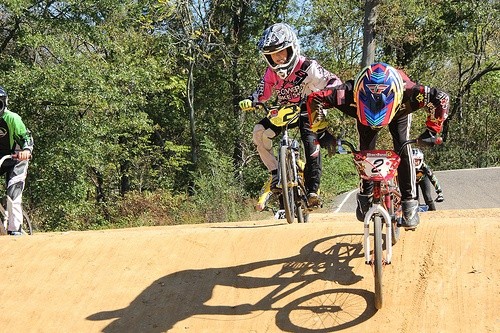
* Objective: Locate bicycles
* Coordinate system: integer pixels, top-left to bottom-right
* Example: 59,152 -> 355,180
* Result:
413,199 -> 444,212
237,97 -> 334,224
321,136 -> 447,309
0,153 -> 33,235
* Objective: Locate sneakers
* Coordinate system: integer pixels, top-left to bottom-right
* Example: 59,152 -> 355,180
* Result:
401,200 -> 420,226
356,193 -> 373,222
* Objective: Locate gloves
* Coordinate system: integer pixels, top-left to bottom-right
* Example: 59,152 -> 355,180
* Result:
239,99 -> 255,112
316,130 -> 337,158
416,129 -> 436,147
434,194 -> 444,203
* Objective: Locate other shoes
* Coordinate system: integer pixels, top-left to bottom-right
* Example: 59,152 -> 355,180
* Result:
270,174 -> 282,194
305,192 -> 320,208
8,231 -> 22,235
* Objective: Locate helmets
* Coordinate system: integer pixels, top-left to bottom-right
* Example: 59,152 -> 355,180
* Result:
0,86 -> 7,106
288,139 -> 300,159
412,148 -> 424,171
257,23 -> 300,80
353,62 -> 403,131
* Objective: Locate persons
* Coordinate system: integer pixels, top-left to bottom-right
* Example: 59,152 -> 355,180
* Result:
0,86 -> 34,235
255,138 -> 320,219
237,24 -> 342,205
301,62 -> 450,226
412,148 -> 444,211
